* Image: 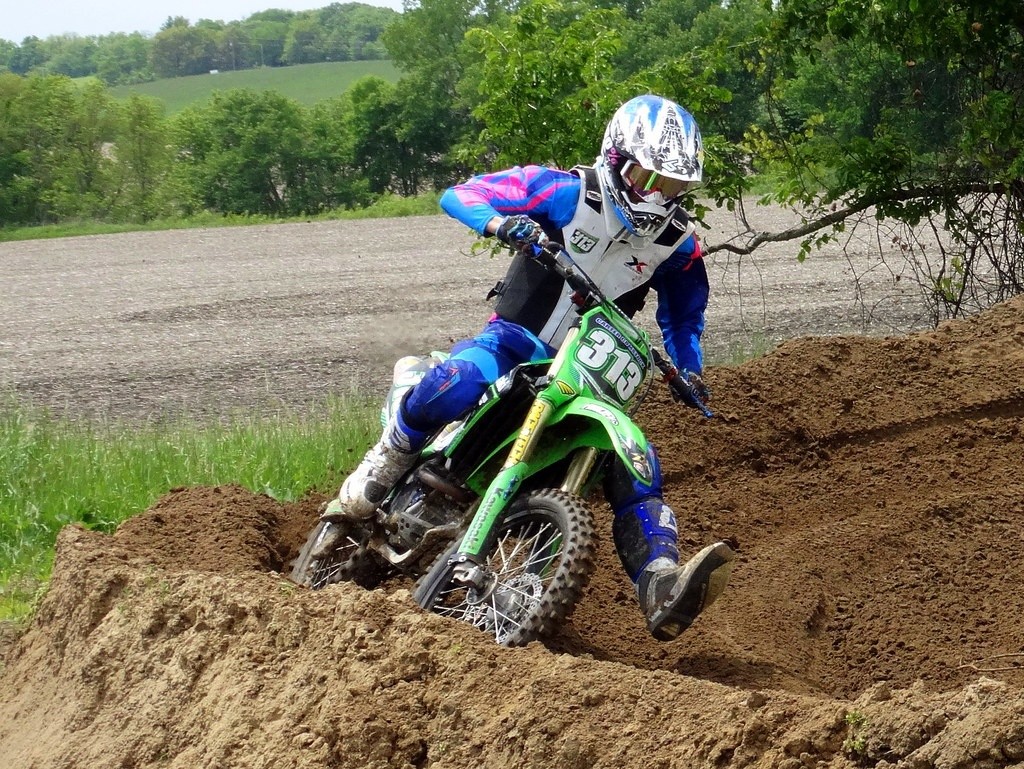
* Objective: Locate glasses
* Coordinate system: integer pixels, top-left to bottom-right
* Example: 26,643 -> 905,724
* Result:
617,160 -> 686,207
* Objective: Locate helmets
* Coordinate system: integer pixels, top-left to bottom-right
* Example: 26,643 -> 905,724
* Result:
596,94 -> 705,235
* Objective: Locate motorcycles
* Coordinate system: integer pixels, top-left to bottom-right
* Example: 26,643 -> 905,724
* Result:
288,215 -> 715,649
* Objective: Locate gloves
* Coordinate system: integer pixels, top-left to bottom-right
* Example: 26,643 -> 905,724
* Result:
496,214 -> 553,259
668,371 -> 709,410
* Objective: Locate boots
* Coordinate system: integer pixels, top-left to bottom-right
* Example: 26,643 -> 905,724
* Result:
611,498 -> 733,641
338,385 -> 445,522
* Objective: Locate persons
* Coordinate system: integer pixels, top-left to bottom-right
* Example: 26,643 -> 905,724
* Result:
339,93 -> 735,641
